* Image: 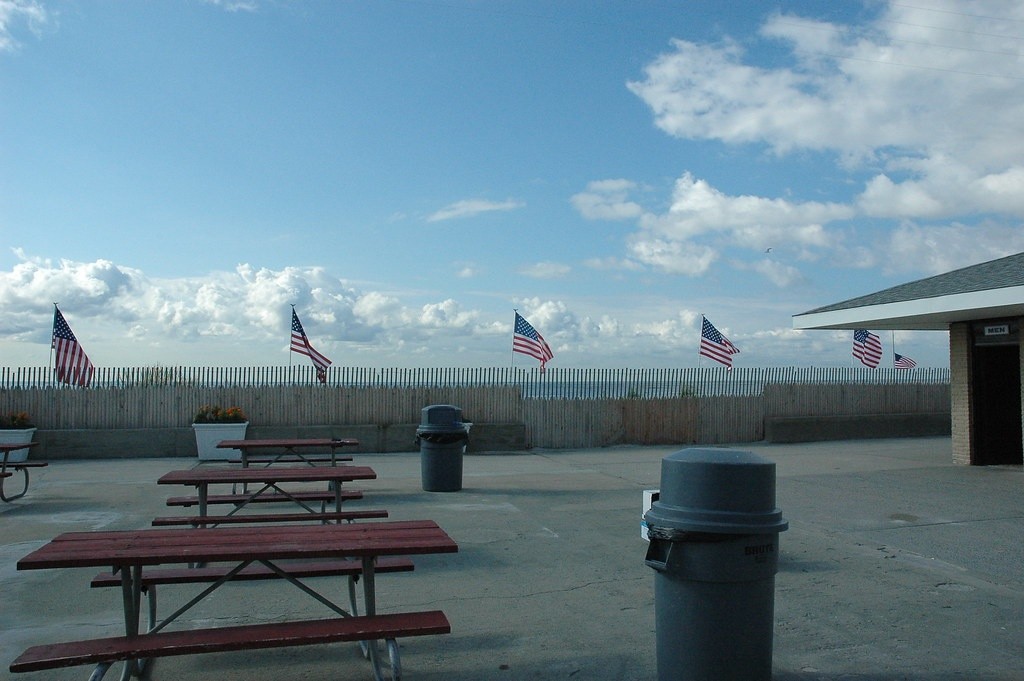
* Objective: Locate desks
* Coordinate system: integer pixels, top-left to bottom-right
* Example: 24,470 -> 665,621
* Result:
152,467 -> 377,570
0,442 -> 40,502
211,438 -> 359,505
17,521 -> 461,681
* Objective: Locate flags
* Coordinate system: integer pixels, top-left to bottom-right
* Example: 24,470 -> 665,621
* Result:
894,352 -> 917,369
52,305 -> 94,388
290,307 -> 332,383
699,316 -> 740,367
852,330 -> 882,369
512,312 -> 554,373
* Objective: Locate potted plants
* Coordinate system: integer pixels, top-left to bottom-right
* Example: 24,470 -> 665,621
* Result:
463,415 -> 473,453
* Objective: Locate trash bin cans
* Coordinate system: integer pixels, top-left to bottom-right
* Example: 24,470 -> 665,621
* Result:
645,448 -> 789,680
417,405 -> 468,492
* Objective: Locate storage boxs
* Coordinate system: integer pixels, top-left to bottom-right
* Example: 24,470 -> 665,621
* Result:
640,488 -> 660,540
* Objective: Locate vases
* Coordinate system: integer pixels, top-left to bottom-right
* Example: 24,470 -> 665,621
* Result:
0,428 -> 37,463
191,421 -> 250,461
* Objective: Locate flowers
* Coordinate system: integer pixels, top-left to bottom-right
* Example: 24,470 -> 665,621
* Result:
0,412 -> 36,430
193,404 -> 247,424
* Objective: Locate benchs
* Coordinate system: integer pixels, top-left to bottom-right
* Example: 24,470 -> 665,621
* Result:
0,462 -> 48,478
166,489 -> 363,507
91,556 -> 414,587
228,456 -> 354,463
152,510 -> 388,526
10,610 -> 451,673
